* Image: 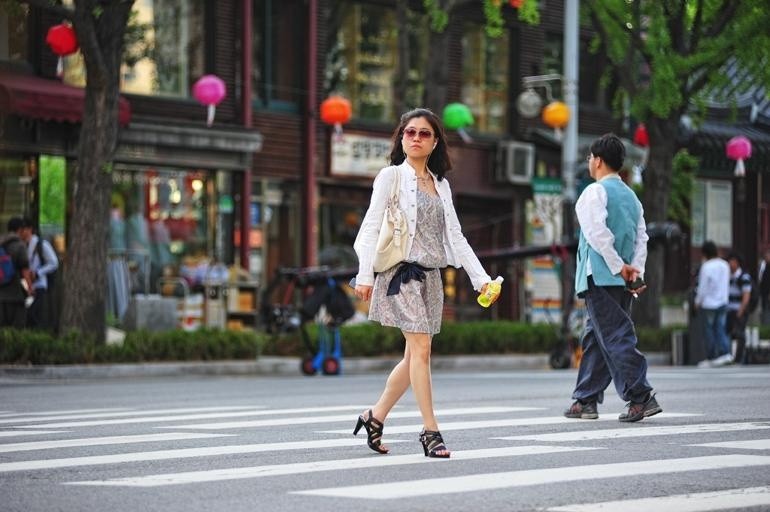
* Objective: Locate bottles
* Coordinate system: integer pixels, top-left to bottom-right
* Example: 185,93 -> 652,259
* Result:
476,275 -> 505,308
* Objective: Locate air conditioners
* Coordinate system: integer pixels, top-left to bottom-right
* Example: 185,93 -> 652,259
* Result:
494,141 -> 536,186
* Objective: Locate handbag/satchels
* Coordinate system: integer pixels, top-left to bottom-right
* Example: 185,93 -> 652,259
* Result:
371,162 -> 409,273
737,271 -> 759,312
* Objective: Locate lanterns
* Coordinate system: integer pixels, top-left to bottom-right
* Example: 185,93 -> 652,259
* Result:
46,18 -> 79,77
725,135 -> 752,176
321,93 -> 353,139
543,101 -> 567,139
444,102 -> 474,146
194,73 -> 227,125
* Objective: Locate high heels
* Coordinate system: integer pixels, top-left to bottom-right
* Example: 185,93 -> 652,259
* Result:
419,428 -> 450,458
353,408 -> 387,453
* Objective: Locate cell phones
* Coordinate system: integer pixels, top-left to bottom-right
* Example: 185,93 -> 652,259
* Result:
629,278 -> 646,290
348,277 -> 356,288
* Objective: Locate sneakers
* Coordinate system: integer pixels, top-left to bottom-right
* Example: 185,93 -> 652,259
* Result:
564,397 -> 598,419
710,353 -> 734,367
697,359 -> 713,369
618,393 -> 662,422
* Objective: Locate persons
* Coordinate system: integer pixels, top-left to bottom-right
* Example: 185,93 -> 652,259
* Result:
109,206 -> 171,278
724,253 -> 752,363
354,108 -> 500,458
564,132 -> 663,422
21,219 -> 58,327
695,242 -> 733,367
0,217 -> 34,327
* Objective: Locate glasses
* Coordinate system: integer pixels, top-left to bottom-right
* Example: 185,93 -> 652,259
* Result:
402,127 -> 432,139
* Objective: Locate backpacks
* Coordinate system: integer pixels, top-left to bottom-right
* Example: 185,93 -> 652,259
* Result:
0,238 -> 18,286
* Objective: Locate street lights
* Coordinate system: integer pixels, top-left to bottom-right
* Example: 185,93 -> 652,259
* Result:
515,69 -> 579,241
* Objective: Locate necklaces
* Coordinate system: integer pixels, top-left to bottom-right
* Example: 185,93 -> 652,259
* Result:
416,173 -> 430,189
